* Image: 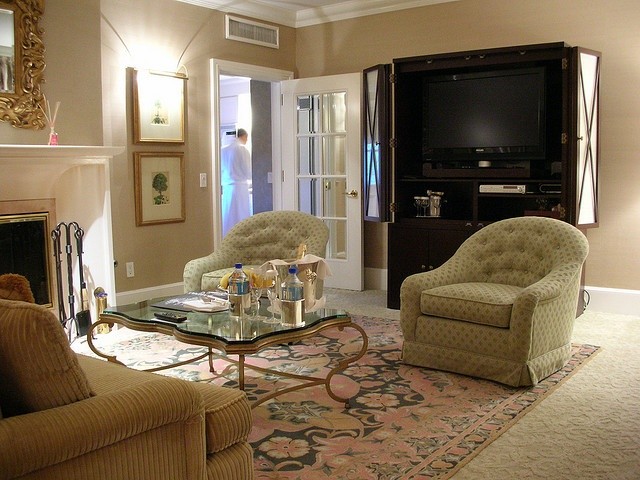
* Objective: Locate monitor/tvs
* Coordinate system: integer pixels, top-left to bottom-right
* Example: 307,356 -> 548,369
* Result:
393,61 -> 549,168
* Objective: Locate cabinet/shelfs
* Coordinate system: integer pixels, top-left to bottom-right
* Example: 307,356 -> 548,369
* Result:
361,40 -> 602,322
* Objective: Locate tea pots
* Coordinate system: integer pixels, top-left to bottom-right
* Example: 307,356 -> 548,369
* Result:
428,192 -> 445,218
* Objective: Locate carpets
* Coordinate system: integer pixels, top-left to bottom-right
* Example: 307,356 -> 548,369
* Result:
80,311 -> 603,479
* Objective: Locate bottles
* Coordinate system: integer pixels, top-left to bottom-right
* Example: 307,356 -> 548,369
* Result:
281,265 -> 306,328
228,263 -> 251,316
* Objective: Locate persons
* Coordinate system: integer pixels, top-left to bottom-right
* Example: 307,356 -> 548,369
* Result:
220,128 -> 252,238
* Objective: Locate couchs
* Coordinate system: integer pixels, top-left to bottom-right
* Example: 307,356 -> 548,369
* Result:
181,210 -> 330,302
1,273 -> 256,479
400,216 -> 590,387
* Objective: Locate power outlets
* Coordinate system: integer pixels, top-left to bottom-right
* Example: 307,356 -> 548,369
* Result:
125,262 -> 135,278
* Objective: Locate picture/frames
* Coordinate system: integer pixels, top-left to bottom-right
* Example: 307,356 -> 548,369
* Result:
127,66 -> 188,148
1,0 -> 46,131
132,149 -> 188,228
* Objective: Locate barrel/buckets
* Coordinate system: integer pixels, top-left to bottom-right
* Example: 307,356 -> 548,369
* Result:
273,258 -> 319,312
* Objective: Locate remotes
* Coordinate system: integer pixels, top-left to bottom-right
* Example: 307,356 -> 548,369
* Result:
154,309 -> 188,324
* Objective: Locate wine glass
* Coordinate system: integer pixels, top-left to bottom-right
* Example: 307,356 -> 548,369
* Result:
263,269 -> 280,324
421,197 -> 429,217
246,268 -> 269,321
414,197 -> 421,217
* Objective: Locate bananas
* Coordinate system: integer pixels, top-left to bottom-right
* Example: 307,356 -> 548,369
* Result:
219,272 -> 229,288
